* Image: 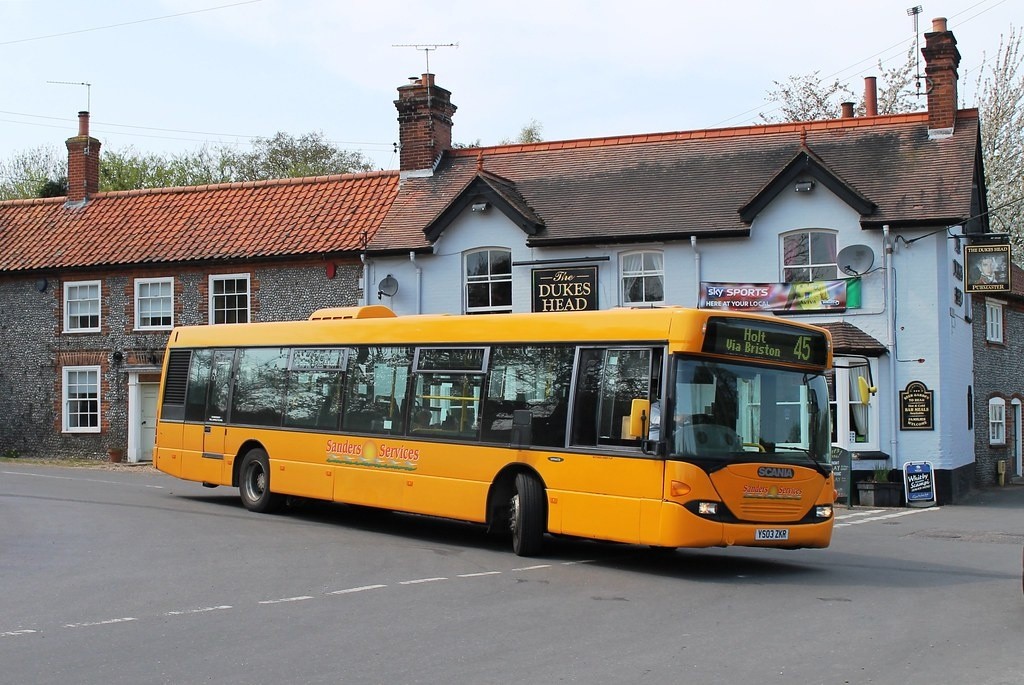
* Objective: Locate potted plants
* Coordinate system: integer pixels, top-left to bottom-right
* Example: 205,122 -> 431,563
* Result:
108,447 -> 126,463
856,464 -> 903,507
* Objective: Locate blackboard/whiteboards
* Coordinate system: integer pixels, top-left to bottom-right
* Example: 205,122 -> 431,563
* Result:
830,447 -> 850,498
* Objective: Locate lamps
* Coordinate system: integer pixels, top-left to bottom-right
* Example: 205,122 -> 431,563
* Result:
472,203 -> 487,212
145,345 -> 156,364
794,179 -> 816,192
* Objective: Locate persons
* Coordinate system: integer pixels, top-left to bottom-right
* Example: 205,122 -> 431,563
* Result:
649,402 -> 688,443
445,412 -> 476,431
414,409 -> 432,430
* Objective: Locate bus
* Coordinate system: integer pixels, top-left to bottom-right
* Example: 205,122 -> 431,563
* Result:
148,305 -> 878,561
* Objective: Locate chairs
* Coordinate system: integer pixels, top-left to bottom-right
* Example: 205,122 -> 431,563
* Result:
350,392 -> 431,432
